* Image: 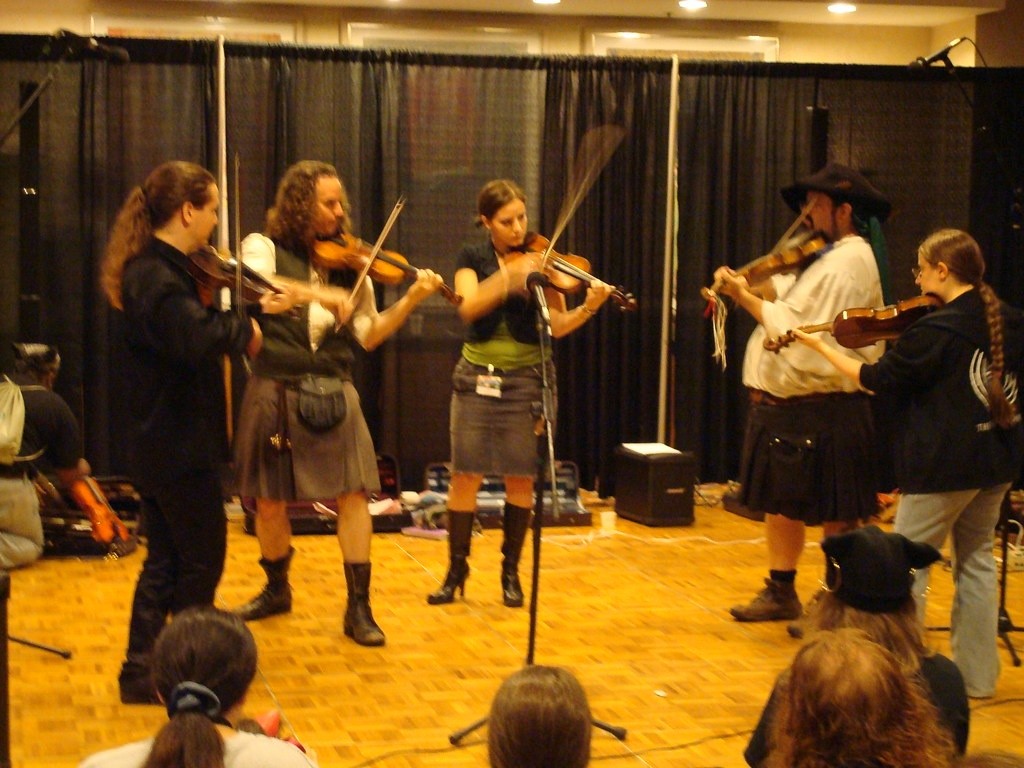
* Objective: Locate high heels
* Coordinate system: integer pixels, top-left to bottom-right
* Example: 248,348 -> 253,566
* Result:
119,652 -> 160,704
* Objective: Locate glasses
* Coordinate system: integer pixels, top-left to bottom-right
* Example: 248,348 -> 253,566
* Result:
911,265 -> 929,279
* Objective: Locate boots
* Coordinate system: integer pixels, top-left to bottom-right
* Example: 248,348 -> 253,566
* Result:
343,560 -> 386,646
238,544 -> 296,620
425,509 -> 475,604
502,500 -> 532,607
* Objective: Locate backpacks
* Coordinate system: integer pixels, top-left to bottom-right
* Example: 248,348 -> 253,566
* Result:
0,374 -> 48,466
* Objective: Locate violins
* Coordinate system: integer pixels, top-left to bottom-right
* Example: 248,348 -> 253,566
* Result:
504,233 -> 637,312
187,245 -> 284,301
763,296 -> 943,354
701,234 -> 826,303
312,230 -> 465,303
69,464 -> 132,544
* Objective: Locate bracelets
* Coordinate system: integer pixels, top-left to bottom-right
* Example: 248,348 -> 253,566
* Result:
580,304 -> 596,316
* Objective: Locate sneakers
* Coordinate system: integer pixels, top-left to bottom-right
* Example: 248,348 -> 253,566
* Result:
729,576 -> 801,621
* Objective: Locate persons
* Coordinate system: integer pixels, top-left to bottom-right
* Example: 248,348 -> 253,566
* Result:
792,229 -> 1024,699
427,180 -> 612,607
233,161 -> 443,647
0,344 -> 90,570
713,162 -> 885,621
488,665 -> 591,768
78,606 -> 317,768
102,159 -> 293,704
744,525 -> 968,768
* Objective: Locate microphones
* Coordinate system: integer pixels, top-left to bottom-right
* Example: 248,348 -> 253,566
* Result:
527,271 -> 552,326
907,37 -> 965,77
57,30 -> 130,64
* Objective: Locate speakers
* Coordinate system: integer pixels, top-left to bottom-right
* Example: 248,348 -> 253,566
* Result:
613,445 -> 695,528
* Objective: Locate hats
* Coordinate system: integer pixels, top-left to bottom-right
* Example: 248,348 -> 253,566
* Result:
821,525 -> 941,616
780,165 -> 892,224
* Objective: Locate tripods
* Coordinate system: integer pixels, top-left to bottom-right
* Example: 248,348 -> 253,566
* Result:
924,52 -> 1024,667
447,315 -> 626,744
